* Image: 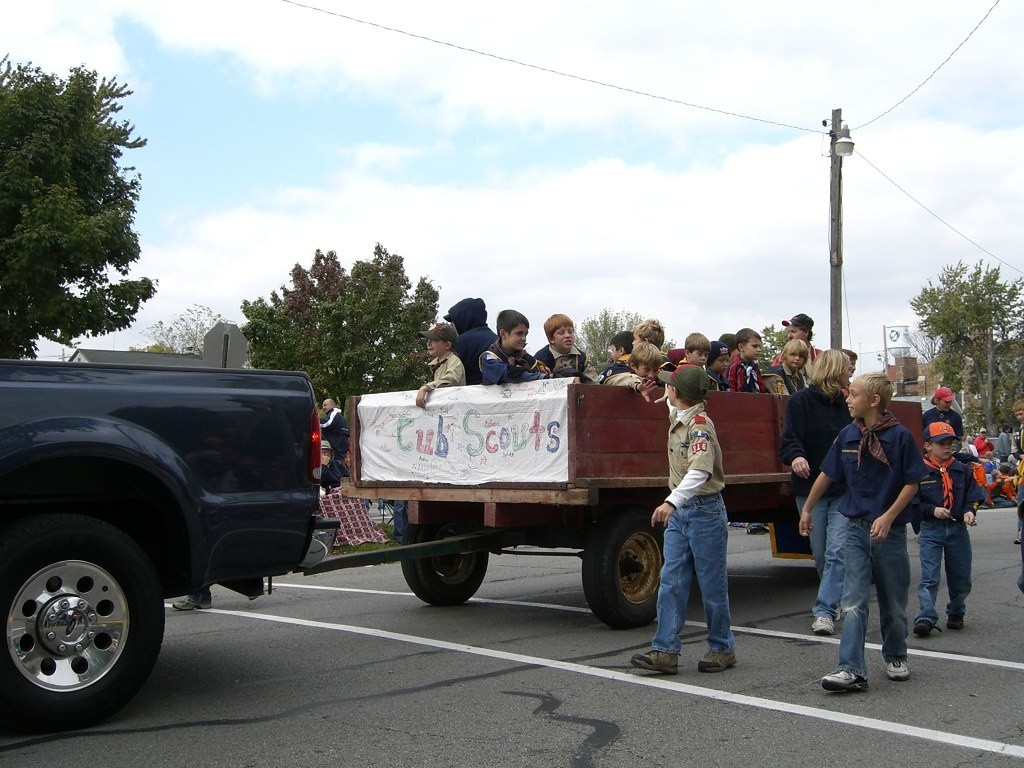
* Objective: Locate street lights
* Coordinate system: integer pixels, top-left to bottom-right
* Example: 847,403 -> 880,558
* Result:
828,107 -> 854,351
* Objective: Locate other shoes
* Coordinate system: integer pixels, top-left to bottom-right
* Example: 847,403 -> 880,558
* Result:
379,508 -> 386,515
1014,538 -> 1020,544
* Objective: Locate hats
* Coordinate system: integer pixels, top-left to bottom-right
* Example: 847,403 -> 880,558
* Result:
417,323 -> 457,347
320,441 -> 335,451
782,313 -> 814,330
443,314 -> 452,322
658,365 -> 710,400
923,421 -> 962,443
705,341 -> 728,366
934,387 -> 955,401
980,427 -> 987,434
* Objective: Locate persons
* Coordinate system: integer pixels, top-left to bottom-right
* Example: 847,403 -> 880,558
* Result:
761,340 -> 808,395
839,349 -> 857,397
781,350 -> 854,635
599,320 -> 765,402
320,399 -> 349,464
416,323 -> 466,408
1013,398 -> 1024,594
772,314 -> 823,386
173,587 -> 259,610
479,309 -> 562,385
909,422 -> 986,637
631,364 -> 738,673
923,387 -> 964,454
799,372 -> 921,691
955,426 -> 1020,509
534,314 -> 599,384
443,298 -> 499,385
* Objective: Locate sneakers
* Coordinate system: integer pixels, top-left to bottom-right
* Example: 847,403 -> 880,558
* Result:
630,650 -> 678,674
811,616 -> 835,635
808,670 -> 869,692
947,615 -> 964,629
886,661 -> 910,681
913,619 -> 942,636
698,649 -> 736,672
835,605 -> 846,621
172,595 -> 212,610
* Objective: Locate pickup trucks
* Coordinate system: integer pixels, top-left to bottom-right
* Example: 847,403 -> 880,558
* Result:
0,357 -> 342,738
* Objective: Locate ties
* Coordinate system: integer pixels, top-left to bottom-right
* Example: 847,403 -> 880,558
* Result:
922,453 -> 956,513
852,410 -> 901,472
